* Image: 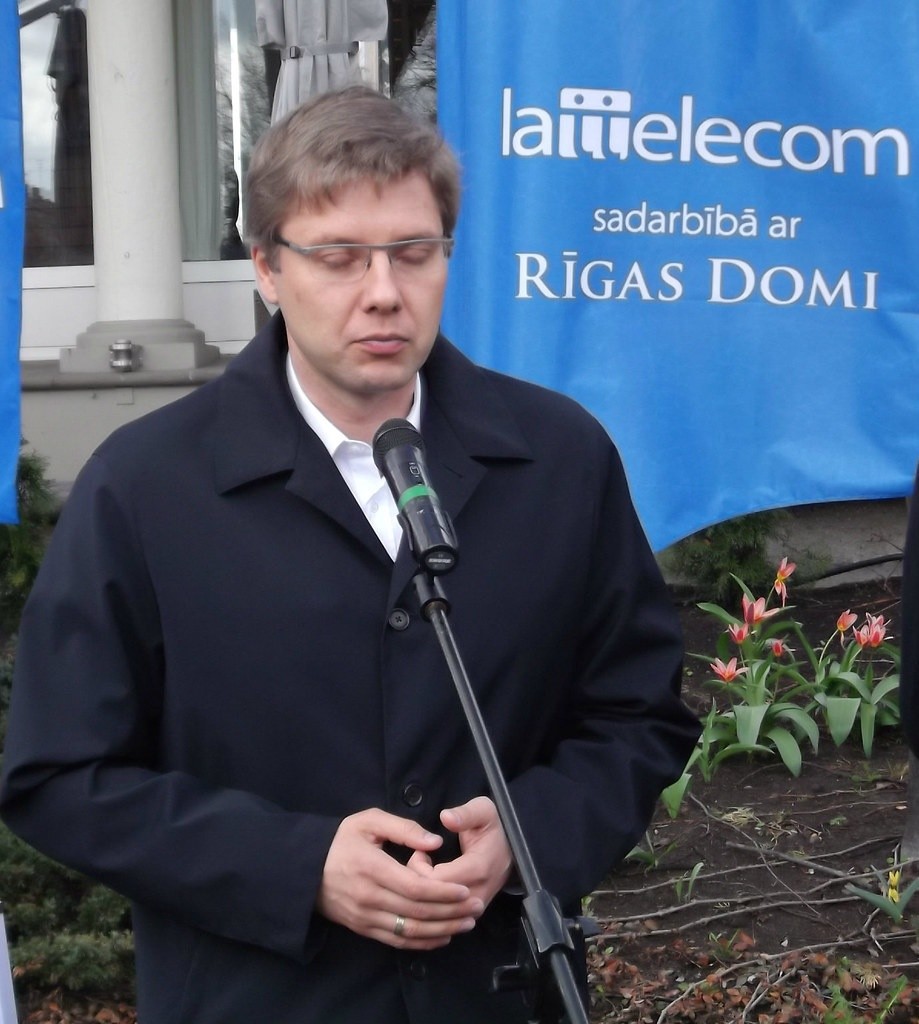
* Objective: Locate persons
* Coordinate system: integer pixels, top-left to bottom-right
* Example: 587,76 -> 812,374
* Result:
0,85 -> 702,1024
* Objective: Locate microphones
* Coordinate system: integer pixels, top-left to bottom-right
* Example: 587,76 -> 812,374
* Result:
371,416 -> 460,578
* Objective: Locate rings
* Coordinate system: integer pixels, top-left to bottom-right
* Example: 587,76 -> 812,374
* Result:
394,915 -> 404,937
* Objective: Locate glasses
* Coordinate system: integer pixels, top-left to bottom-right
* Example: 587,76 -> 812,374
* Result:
274,234 -> 456,284
